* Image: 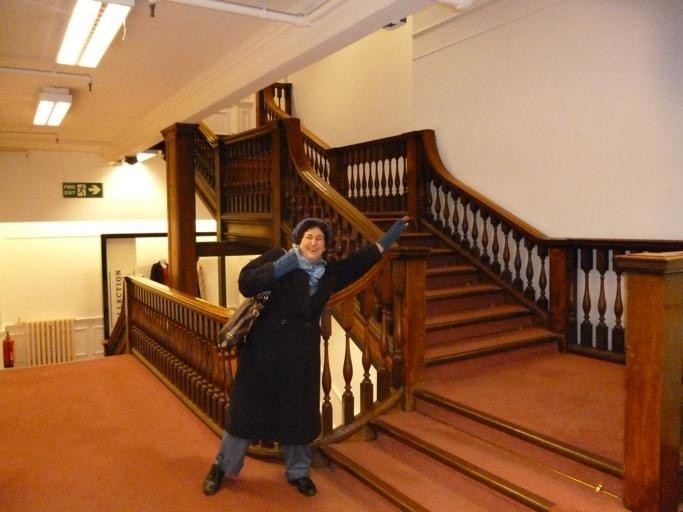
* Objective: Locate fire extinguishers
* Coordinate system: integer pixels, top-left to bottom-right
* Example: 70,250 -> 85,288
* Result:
3,329 -> 14,368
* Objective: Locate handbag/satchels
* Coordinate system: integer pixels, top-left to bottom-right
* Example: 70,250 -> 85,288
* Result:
216,297 -> 266,348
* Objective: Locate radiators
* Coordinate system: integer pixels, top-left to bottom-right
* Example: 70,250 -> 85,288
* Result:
26,316 -> 77,366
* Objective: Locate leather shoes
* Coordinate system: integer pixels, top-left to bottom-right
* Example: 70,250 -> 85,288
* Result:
293,480 -> 316,495
204,463 -> 224,495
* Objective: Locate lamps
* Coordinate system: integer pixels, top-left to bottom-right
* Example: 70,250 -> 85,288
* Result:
34,86 -> 73,129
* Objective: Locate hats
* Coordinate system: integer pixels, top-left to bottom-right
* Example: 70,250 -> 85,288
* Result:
290,218 -> 332,246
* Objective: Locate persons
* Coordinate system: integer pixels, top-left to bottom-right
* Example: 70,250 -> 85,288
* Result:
151,258 -> 169,286
204,214 -> 410,495
